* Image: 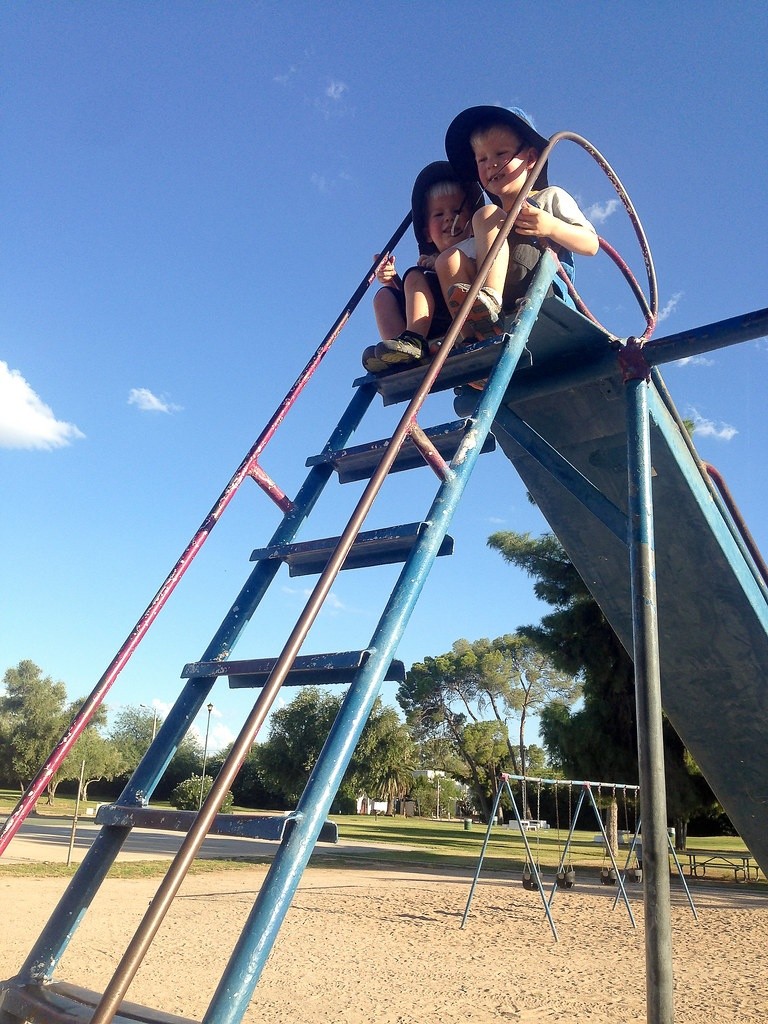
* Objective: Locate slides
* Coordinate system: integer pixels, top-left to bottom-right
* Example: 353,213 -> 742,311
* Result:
457,298 -> 767,1024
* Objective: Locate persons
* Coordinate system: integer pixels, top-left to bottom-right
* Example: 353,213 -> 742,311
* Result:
360,105 -> 599,391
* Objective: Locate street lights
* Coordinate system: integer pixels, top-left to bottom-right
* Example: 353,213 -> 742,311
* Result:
139,703 -> 157,743
199,702 -> 214,812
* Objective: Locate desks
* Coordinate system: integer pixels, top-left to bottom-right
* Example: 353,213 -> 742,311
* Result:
676,848 -> 760,883
529,820 -> 547,829
616,830 -> 630,843
509,820 -> 530,829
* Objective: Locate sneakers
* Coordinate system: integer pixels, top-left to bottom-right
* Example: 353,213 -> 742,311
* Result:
363,342 -> 389,372
427,320 -> 460,355
448,283 -> 505,342
376,328 -> 427,363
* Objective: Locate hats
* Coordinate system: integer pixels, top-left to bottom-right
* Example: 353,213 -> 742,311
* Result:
412,160 -> 487,253
445,105 -> 549,191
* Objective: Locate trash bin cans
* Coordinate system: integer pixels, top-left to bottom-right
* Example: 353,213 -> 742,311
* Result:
464,818 -> 472,830
668,828 -> 675,837
493,816 -> 498,827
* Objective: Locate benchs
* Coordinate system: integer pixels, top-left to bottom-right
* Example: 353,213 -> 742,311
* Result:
524,826 -> 537,831
86,807 -> 94,815
673,863 -> 746,870
530,824 -> 541,829
696,861 -> 760,869
